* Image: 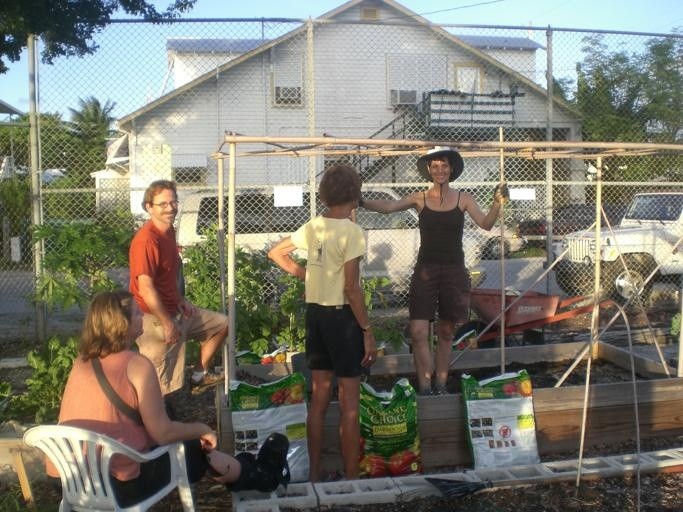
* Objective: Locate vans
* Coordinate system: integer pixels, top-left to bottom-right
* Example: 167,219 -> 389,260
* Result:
176,188 -> 479,305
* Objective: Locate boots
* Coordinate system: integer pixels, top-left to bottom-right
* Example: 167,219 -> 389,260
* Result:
225,432 -> 290,492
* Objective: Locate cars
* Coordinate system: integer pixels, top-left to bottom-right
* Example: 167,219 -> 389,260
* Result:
463,204 -> 627,259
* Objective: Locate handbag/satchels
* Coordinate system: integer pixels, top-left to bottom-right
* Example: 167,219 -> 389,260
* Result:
149,436 -> 207,485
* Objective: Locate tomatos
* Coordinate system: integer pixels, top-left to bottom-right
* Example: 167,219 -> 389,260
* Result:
361,453 -> 388,477
389,450 -> 417,475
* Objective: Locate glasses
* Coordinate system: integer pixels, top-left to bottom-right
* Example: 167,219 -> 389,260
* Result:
153,200 -> 180,208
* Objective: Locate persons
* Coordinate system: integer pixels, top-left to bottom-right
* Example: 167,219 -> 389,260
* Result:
128,180 -> 230,400
359,148 -> 509,397
46,291 -> 289,508
267,165 -> 377,481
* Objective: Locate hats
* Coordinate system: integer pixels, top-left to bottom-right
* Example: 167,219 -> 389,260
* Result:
416,145 -> 463,182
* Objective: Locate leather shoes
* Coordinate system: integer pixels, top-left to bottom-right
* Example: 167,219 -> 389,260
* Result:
191,369 -> 225,396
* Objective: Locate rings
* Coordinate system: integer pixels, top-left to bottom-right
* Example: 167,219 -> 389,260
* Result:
369,356 -> 375,362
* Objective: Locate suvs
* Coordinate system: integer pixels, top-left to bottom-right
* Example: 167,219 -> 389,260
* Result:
543,193 -> 683,302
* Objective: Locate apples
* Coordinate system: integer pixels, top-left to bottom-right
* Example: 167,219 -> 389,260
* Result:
271,384 -> 305,404
502,379 -> 532,396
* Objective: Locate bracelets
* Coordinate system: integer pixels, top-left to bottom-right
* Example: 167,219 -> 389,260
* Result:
361,324 -> 372,331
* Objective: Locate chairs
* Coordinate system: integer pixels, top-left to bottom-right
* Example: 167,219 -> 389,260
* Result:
24,426 -> 198,510
